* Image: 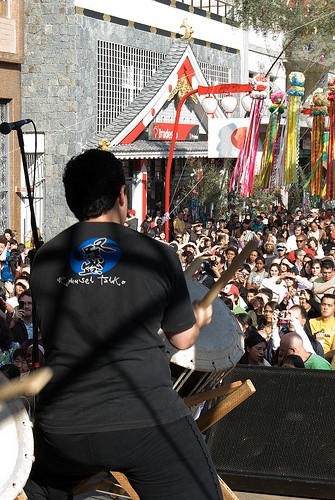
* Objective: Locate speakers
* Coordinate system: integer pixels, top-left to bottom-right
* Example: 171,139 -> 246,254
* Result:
206,366 -> 334,500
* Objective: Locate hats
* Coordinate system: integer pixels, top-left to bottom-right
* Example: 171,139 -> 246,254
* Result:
128,209 -> 136,215
219,284 -> 240,299
27,343 -> 44,357
309,209 -> 318,215
277,242 -> 287,251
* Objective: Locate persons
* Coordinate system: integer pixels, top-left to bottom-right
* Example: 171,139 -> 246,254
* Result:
24,149 -> 225,500
0,201 -> 335,425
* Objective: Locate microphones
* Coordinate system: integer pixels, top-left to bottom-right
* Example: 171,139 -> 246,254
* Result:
0,119 -> 30,135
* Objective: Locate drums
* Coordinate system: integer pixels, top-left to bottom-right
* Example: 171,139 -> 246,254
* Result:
157,273 -> 245,421
0,373 -> 35,499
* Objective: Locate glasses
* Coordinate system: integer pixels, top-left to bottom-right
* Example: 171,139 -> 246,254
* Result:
176,236 -> 182,239
263,310 -> 274,313
315,221 -> 320,223
296,239 -> 306,243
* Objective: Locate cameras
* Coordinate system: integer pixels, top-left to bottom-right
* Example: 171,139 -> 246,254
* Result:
209,260 -> 218,265
278,311 -> 285,317
